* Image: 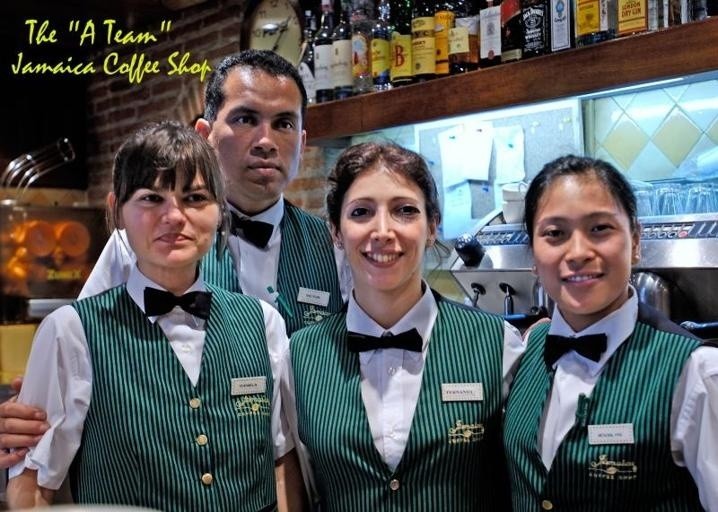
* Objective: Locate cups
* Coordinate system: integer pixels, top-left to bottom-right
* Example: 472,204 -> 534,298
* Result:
622,185 -> 715,217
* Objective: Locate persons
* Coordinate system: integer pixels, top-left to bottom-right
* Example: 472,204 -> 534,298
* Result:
5,123 -> 305,511
1,50 -> 354,480
500,154 -> 717,512
280,141 -> 524,511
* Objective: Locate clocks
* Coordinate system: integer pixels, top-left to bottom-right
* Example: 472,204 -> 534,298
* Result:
239,1 -> 304,74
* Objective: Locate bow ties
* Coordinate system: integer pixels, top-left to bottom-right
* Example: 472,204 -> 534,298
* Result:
346,328 -> 423,353
544,331 -> 609,373
143,286 -> 212,320
227,210 -> 275,248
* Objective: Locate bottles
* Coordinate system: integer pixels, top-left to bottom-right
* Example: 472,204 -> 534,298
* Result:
294,0 -> 715,114
